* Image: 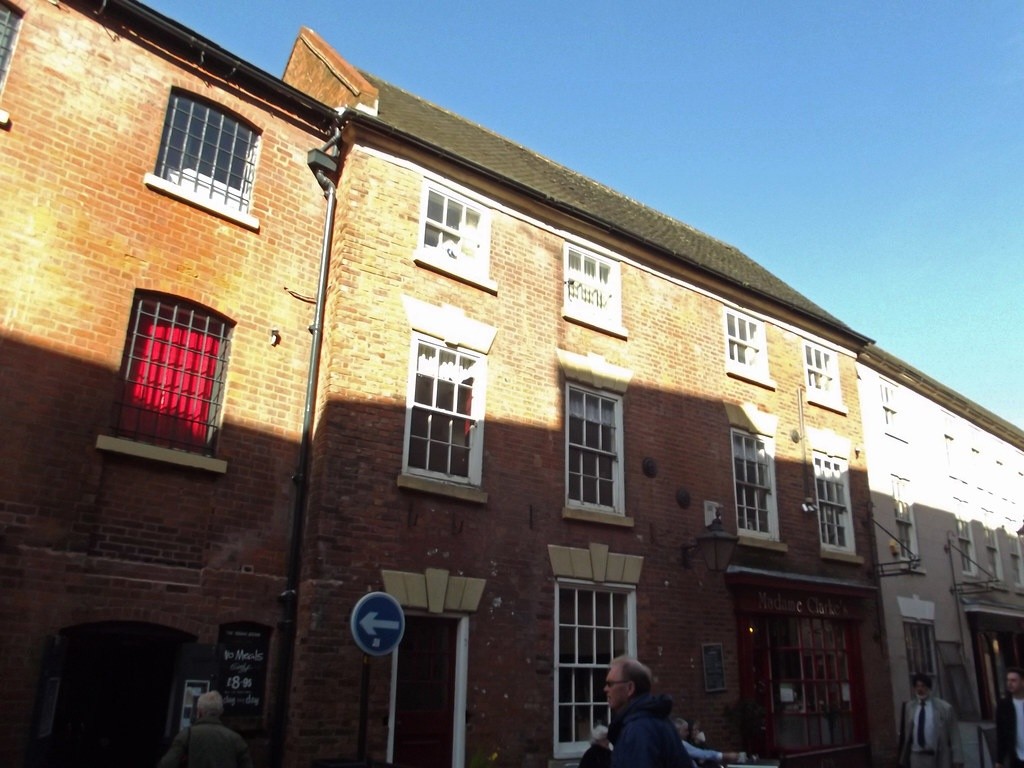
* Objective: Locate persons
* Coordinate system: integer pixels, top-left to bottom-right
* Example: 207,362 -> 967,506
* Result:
156,690 -> 253,768
578,655 -> 749,768
897,672 -> 965,768
994,667 -> 1024,768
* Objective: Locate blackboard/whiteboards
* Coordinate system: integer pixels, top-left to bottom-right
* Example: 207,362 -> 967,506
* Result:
216,621 -> 270,716
701,643 -> 727,692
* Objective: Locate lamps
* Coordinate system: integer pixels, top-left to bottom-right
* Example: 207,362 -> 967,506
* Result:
681,507 -> 741,575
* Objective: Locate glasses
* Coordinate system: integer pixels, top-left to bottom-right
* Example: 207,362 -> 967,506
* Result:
607,680 -> 629,687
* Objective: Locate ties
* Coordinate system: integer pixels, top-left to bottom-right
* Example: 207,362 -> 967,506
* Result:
917,702 -> 928,748
